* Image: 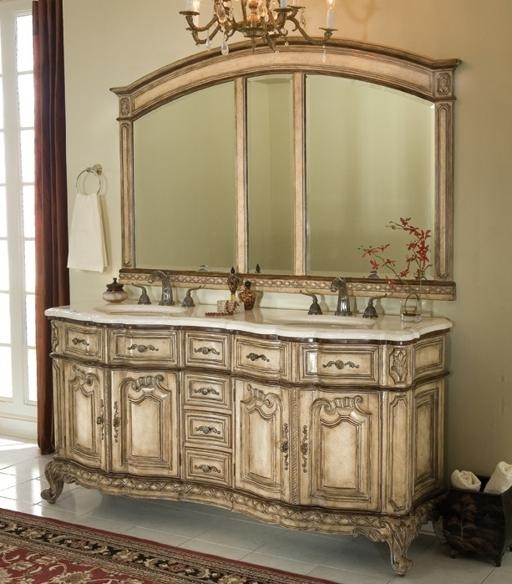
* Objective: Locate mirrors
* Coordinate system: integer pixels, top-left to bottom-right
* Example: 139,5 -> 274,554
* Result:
104,35 -> 459,304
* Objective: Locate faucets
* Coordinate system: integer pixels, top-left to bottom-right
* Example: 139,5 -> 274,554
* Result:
148,270 -> 172,304
367,268 -> 380,277
198,264 -> 210,271
329,280 -> 354,316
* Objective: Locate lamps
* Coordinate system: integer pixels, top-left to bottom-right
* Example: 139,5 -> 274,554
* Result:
180,1 -> 343,56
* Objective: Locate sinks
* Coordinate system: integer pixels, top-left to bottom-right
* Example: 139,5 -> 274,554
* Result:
269,313 -> 378,335
95,304 -> 188,319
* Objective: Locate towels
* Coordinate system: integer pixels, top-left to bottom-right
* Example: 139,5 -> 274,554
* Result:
482,458 -> 512,496
63,191 -> 110,276
448,466 -> 486,492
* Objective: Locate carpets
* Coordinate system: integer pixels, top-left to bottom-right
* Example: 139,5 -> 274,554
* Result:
0,501 -> 345,584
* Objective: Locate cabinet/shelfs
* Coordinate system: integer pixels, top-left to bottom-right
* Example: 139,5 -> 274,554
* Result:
38,307 -> 172,507
172,307 -> 230,511
227,308 -> 454,578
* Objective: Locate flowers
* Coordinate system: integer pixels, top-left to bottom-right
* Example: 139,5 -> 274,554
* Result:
361,208 -> 443,313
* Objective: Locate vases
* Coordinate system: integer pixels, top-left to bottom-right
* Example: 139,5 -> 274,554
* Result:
401,286 -> 424,323
